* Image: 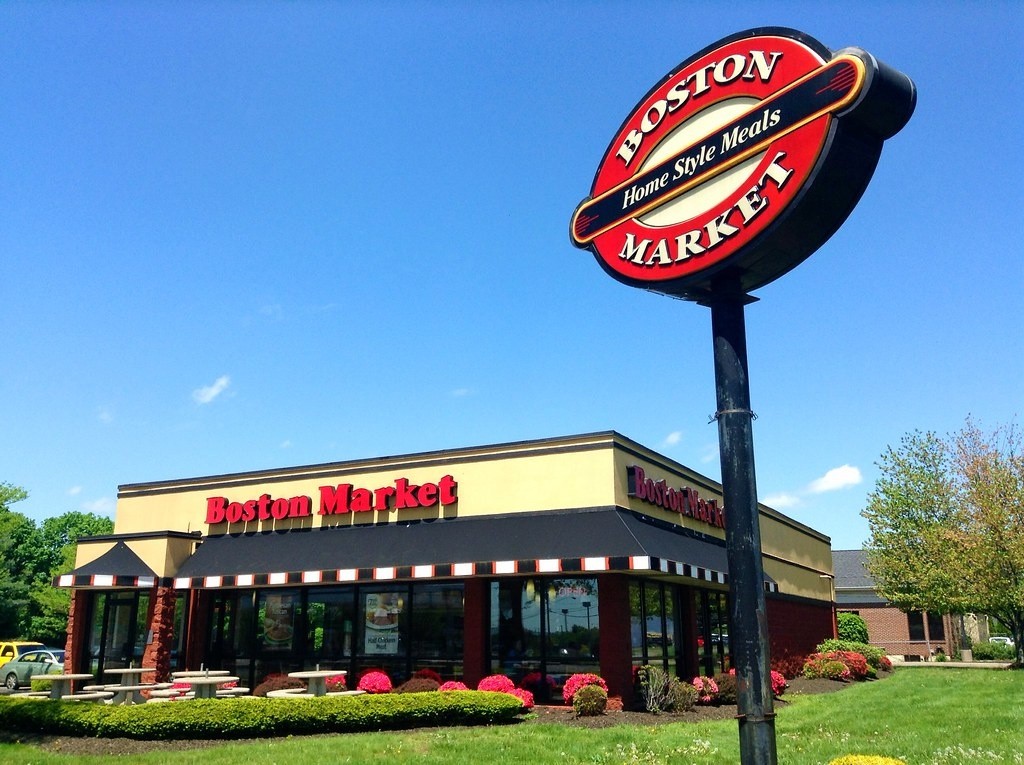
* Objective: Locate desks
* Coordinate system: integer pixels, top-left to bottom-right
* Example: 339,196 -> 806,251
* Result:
104,668 -> 157,706
171,671 -> 230,691
288,670 -> 347,697
30,674 -> 94,699
172,676 -> 240,699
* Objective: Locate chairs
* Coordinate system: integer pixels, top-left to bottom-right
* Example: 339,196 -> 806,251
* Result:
41,657 -> 47,662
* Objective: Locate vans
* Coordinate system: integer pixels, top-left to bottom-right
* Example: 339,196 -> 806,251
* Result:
0,641 -> 49,669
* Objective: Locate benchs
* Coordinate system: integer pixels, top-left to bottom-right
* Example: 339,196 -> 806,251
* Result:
10,684 -> 250,705
266,688 -> 315,698
327,690 -> 367,697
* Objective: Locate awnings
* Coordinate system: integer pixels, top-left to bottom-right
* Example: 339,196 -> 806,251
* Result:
52,542 -> 159,587
175,508 -> 777,592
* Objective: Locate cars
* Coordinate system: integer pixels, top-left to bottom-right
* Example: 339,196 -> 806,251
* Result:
0,649 -> 66,690
989,636 -> 1016,650
